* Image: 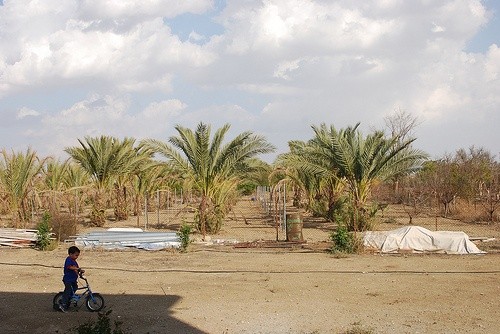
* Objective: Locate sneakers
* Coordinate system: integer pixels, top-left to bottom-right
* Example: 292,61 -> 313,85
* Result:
59,305 -> 65,312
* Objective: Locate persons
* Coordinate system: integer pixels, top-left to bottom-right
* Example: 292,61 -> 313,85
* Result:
59,246 -> 85,313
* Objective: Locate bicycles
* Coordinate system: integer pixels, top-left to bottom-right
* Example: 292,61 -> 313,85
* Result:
52,269 -> 104,312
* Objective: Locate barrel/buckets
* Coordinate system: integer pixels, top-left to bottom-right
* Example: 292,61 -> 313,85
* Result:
286,214 -> 304,241
